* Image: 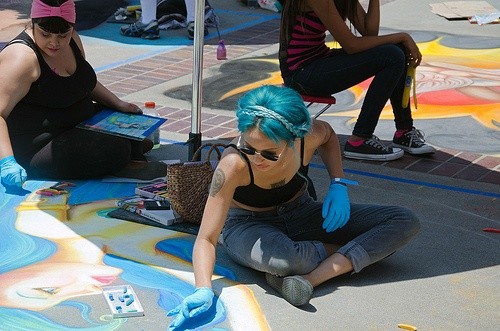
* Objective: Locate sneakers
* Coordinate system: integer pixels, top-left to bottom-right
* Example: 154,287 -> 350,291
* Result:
343,135 -> 404,161
120,19 -> 159,38
188,21 -> 208,39
392,127 -> 435,154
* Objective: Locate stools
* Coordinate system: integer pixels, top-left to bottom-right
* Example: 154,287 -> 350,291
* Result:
301,94 -> 336,119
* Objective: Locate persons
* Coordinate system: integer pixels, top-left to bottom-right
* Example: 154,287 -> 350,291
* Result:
0,0 -> 156,188
278,0 -> 435,160
166,85 -> 421,330
120,0 -> 207,40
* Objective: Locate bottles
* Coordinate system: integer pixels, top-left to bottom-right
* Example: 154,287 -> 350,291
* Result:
216,39 -> 226,60
142,102 -> 161,149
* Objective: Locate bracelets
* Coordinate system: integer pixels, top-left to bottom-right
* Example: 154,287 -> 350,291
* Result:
329,178 -> 360,186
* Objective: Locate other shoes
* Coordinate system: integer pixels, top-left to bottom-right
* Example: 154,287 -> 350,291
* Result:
265,272 -> 313,307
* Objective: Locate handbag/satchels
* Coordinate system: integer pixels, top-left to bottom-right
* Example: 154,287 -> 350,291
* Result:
166,143 -> 226,223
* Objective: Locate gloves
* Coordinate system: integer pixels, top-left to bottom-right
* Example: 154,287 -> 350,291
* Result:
167,288 -> 215,328
0,156 -> 27,187
321,178 -> 359,233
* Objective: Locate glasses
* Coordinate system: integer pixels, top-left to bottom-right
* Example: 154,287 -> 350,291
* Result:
236,136 -> 287,162
